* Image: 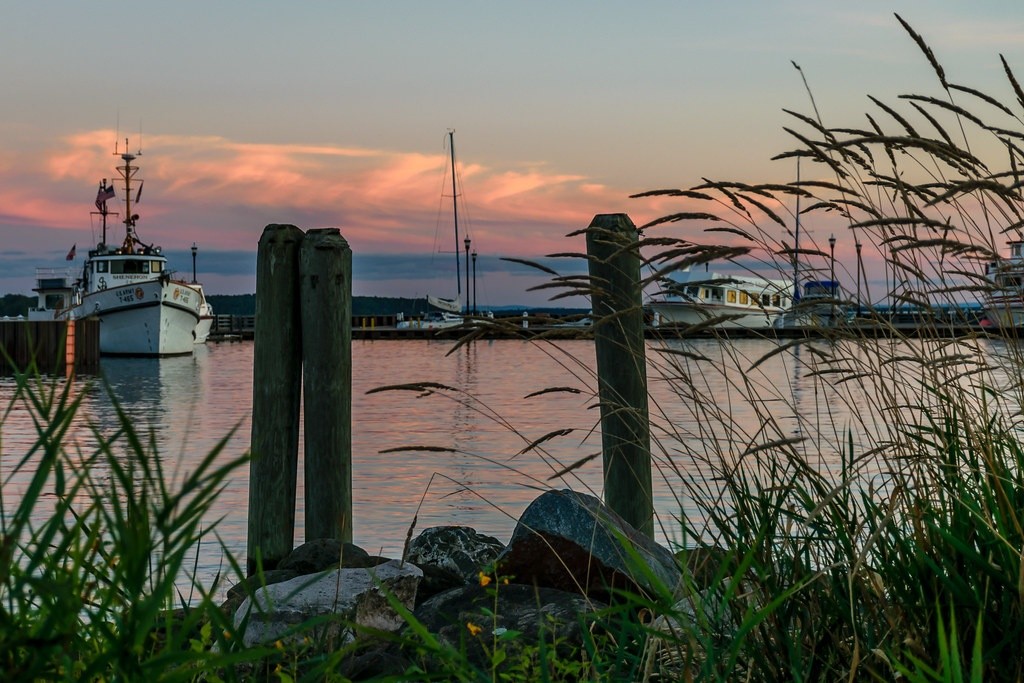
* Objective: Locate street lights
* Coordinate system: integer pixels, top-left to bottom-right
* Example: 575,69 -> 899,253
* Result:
464,236 -> 471,327
191,241 -> 197,285
855,239 -> 862,318
828,234 -> 838,331
470,250 -> 477,320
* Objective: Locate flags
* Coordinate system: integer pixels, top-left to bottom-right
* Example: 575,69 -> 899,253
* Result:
98,185 -> 115,202
95,185 -> 102,211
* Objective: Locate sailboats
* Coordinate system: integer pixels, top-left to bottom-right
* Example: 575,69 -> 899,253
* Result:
397,128 -> 499,328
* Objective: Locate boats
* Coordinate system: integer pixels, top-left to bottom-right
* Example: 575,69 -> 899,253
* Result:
648,268 -> 803,330
775,275 -> 857,333
983,236 -> 1024,327
52,139 -> 203,356
182,283 -> 217,345
